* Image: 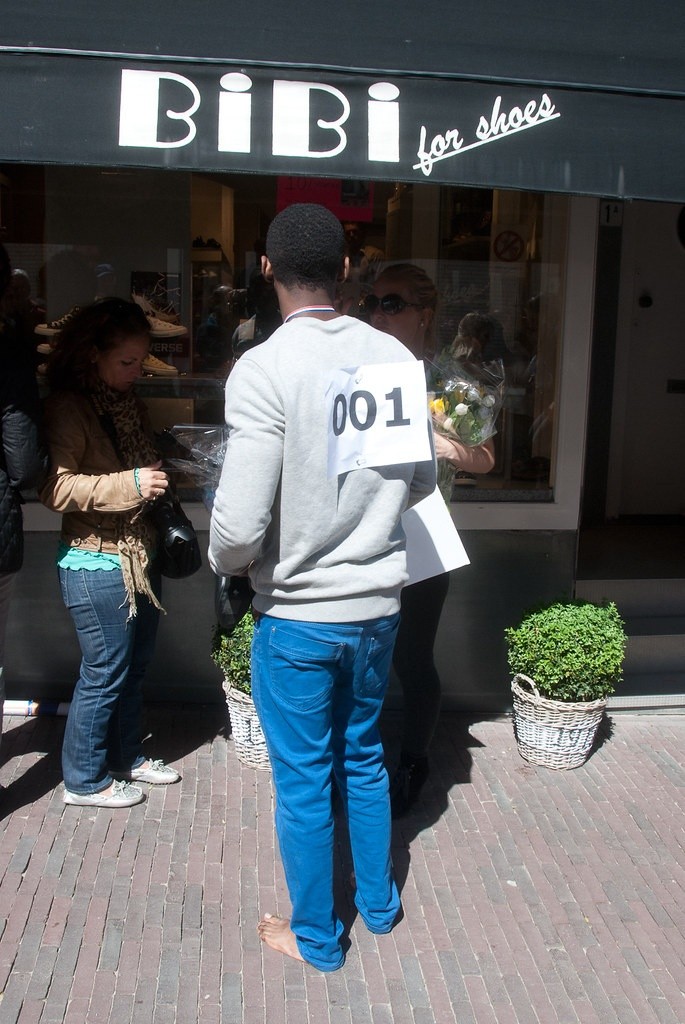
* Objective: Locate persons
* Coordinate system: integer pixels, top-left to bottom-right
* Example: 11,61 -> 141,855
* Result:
197,286 -> 240,367
93,263 -> 119,296
42,297 -> 181,808
230,266 -> 283,370
0,244 -> 41,708
332,263 -> 495,822
450,296 -> 539,390
342,221 -> 384,312
0,269 -> 45,322
208,205 -> 438,972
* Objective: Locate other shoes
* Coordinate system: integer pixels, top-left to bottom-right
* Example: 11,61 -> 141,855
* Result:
62,778 -> 144,808
107,757 -> 182,786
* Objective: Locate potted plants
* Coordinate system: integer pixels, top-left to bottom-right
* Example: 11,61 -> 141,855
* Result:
504,595 -> 627,768
210,601 -> 273,772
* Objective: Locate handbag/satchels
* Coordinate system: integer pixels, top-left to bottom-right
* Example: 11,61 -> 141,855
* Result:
145,490 -> 203,581
213,575 -> 251,629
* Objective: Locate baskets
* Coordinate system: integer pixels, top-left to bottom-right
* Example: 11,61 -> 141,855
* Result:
222,679 -> 274,773
509,673 -> 610,771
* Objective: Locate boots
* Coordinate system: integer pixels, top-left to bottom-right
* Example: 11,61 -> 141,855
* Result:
388,753 -> 429,820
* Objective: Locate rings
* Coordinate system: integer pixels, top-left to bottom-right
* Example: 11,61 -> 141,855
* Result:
156,488 -> 161,496
152,496 -> 155,501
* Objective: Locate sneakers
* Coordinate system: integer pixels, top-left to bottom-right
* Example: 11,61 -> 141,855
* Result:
140,353 -> 179,376
35,343 -> 58,356
148,315 -> 189,340
34,304 -> 84,336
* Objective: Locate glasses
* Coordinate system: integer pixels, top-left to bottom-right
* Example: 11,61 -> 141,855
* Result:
363,293 -> 426,317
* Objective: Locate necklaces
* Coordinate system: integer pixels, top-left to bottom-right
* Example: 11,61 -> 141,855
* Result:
284,305 -> 334,323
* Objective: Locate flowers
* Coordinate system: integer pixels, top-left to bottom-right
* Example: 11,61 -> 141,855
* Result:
426,365 -> 497,495
